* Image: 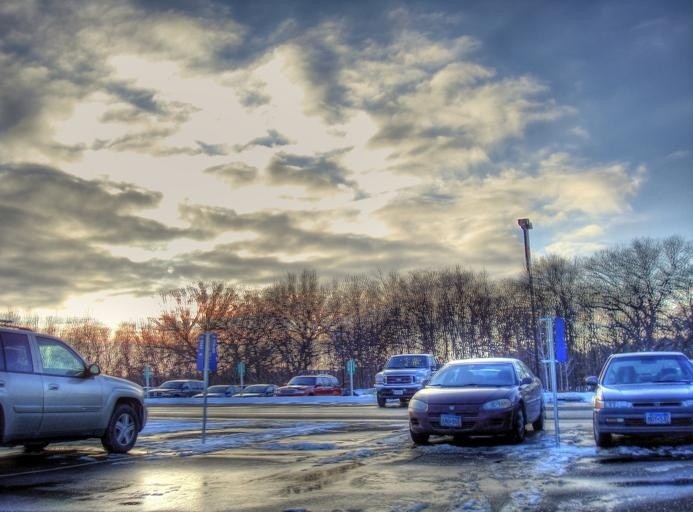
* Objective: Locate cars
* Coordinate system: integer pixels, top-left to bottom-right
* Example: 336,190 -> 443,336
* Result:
193,384 -> 243,397
586,352 -> 693,446
233,384 -> 280,396
408,358 -> 545,444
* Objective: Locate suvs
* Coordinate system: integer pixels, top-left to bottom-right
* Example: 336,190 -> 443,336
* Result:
374,353 -> 437,406
277,374 -> 341,395
145,379 -> 203,398
0,319 -> 149,454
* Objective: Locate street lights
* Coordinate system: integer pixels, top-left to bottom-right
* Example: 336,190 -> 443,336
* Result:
519,218 -> 539,377
